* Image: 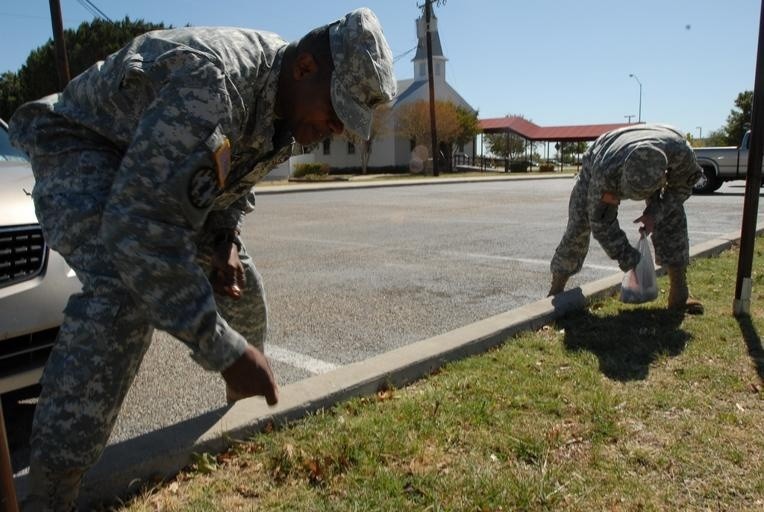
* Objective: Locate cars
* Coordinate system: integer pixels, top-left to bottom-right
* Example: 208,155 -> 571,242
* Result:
0,120 -> 83,406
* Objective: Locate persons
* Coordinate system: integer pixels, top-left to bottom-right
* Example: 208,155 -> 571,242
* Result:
547,122 -> 705,314
7,5 -> 403,512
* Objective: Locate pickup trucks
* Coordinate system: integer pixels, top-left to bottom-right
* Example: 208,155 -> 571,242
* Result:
687,128 -> 764,195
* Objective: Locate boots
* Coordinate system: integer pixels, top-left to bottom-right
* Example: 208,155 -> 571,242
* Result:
547,274 -> 568,296
29,457 -> 81,512
667,264 -> 704,313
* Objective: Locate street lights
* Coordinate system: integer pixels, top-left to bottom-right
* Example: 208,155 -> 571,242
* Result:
629,74 -> 642,124
624,115 -> 636,123
695,127 -> 701,138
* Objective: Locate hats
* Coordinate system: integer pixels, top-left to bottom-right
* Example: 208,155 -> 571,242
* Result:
620,144 -> 667,200
328,7 -> 395,140
